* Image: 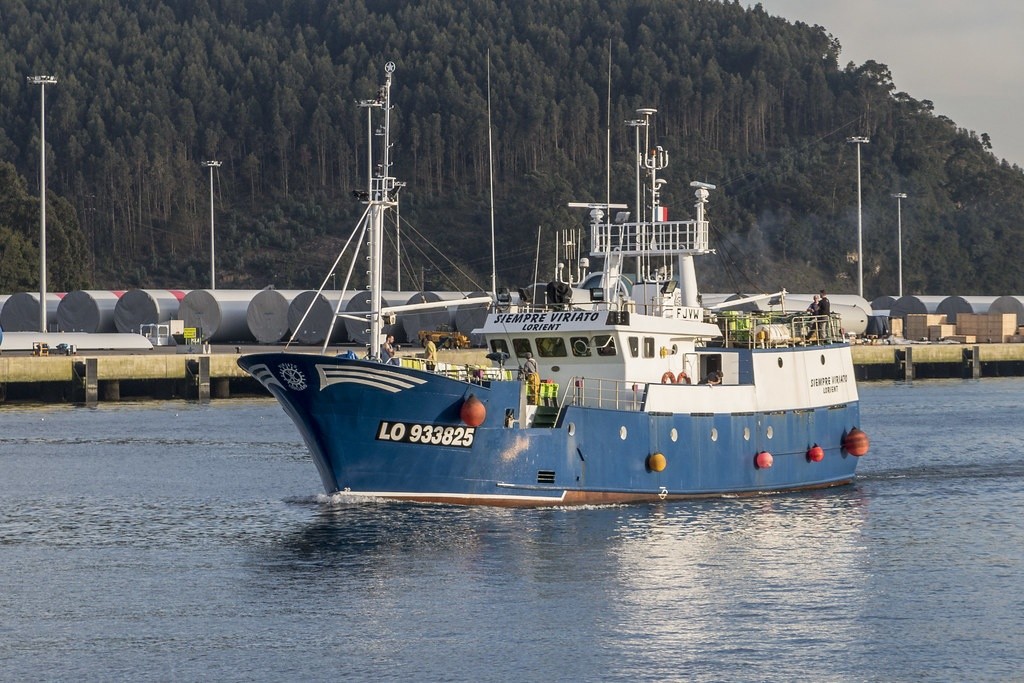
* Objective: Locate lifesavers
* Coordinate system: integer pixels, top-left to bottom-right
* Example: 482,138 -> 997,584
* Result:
677,372 -> 691,384
661,371 -> 678,385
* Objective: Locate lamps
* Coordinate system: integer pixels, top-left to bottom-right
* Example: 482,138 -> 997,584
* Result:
660,281 -> 677,294
590,288 -> 604,302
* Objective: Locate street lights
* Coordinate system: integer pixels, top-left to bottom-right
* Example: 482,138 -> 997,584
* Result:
200,161 -> 223,288
26,75 -> 58,333
890,192 -> 908,297
845,136 -> 870,297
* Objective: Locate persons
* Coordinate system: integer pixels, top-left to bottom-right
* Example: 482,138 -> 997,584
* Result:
802,289 -> 830,343
698,372 -> 721,385
520,352 -> 540,404
423,335 -> 437,373
381,335 -> 399,365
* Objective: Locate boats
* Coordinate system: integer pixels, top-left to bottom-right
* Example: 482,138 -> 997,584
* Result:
236,37 -> 863,508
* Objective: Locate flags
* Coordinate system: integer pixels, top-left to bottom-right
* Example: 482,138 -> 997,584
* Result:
647,207 -> 667,221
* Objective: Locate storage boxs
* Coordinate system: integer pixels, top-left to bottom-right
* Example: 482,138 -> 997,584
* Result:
890,312 -> 1024,342
